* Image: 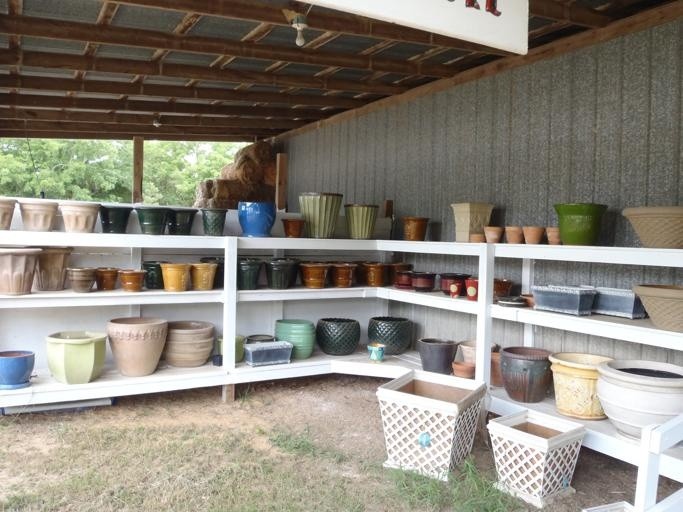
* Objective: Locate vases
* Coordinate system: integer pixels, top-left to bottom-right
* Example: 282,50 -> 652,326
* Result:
385,261 -> 478,302
237,248 -> 385,291
0,244 -> 224,297
398,216 -> 430,242
235,300 -> 477,379
486,409 -> 587,510
449,201 -> 682,250
375,368 -> 488,482
414,334 -> 682,442
492,276 -> 683,334
0,315 -> 248,392
0,189 -> 380,241
